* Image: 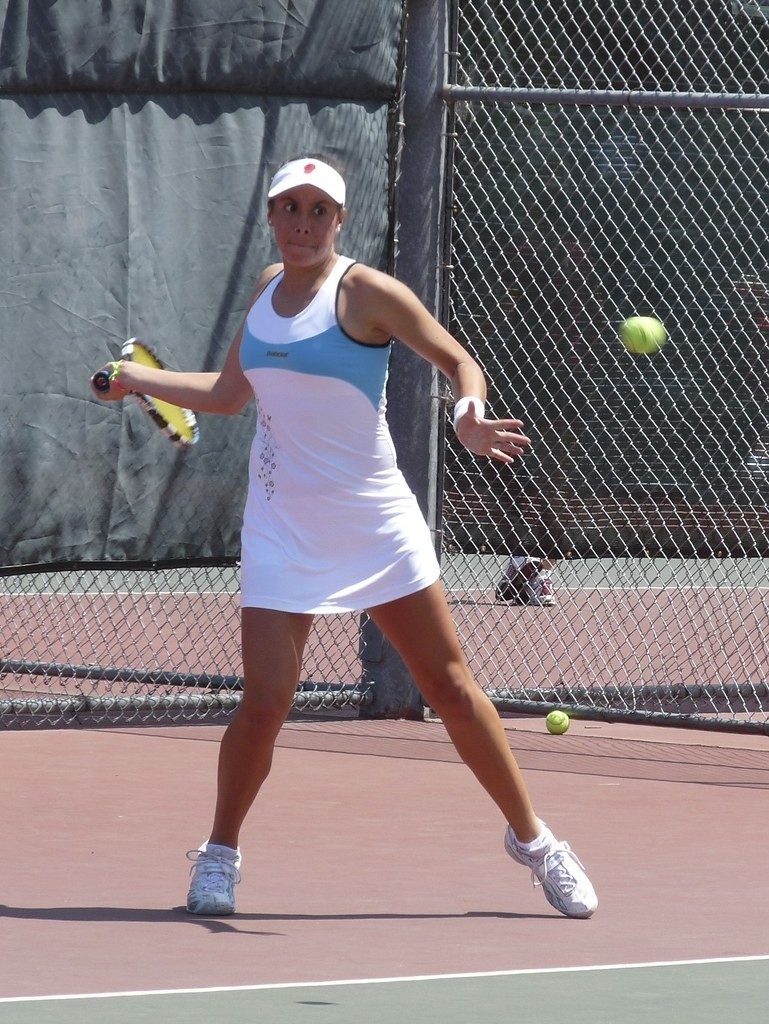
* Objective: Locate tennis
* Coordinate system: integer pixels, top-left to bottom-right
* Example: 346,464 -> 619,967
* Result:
621,316 -> 668,354
545,710 -> 571,735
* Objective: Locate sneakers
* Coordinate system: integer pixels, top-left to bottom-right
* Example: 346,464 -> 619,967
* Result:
504,815 -> 599,919
185,838 -> 242,915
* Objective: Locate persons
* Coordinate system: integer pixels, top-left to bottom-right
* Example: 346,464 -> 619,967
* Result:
92,154 -> 598,919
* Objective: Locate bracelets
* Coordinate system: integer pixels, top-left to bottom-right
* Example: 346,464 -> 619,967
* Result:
110,360 -> 132,393
453,396 -> 484,432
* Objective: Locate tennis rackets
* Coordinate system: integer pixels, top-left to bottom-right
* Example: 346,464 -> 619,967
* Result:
92,336 -> 200,451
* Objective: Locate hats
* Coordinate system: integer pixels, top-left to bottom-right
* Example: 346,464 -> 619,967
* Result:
267,158 -> 346,208
